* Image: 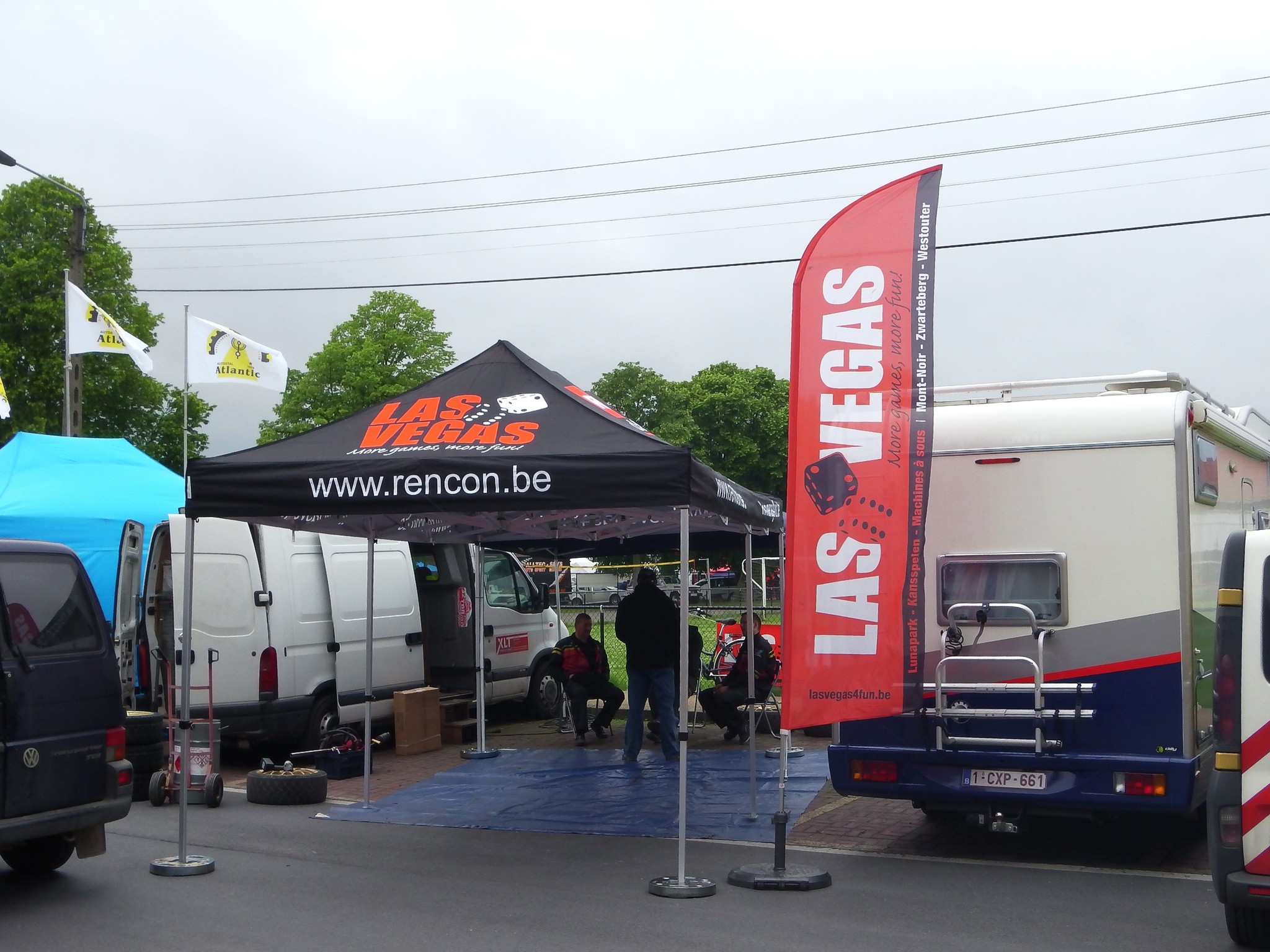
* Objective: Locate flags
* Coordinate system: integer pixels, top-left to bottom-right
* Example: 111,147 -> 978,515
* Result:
779,164 -> 950,735
66,281 -> 153,374
0,375 -> 12,420
187,313 -> 288,394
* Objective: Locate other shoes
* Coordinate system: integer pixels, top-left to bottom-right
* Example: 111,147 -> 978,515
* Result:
665,755 -> 680,761
576,735 -> 586,746
622,754 -> 637,761
646,719 -> 662,743
590,723 -> 608,739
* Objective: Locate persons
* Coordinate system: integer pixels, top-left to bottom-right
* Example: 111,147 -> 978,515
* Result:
615,568 -> 682,761
642,609 -> 704,744
550,613 -> 626,747
698,612 -> 778,744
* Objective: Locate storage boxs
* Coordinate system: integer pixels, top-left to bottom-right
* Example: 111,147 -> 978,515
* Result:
393,686 -> 443,756
314,751 -> 373,781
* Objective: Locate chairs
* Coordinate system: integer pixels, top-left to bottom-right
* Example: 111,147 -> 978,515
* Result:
558,687 -> 614,741
726,659 -> 782,746
676,657 -> 707,734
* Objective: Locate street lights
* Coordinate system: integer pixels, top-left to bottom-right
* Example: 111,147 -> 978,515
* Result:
0,150 -> 87,438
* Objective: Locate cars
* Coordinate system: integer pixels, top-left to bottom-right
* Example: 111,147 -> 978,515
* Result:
568,575 -> 738,609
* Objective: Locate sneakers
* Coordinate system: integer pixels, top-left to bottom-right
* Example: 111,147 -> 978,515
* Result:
724,724 -> 738,741
738,719 -> 756,745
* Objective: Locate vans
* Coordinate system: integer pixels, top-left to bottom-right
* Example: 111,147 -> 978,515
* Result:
1,540 -> 134,873
1207,529 -> 1270,952
114,515 -> 571,764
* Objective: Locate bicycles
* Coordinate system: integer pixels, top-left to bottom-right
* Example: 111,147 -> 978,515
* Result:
687,608 -> 745,694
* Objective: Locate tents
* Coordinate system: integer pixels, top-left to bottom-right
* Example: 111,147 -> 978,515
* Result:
2,430 -> 189,711
174,339 -> 786,904
703,563 -> 743,577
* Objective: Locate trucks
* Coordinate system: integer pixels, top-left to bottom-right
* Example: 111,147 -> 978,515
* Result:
828,370 -> 1270,863
